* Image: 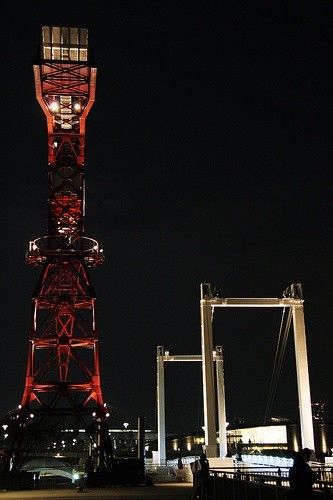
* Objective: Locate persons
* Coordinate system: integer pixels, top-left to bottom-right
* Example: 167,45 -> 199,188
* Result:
190,453 -> 211,499
292,448 -> 320,499
85,456 -> 95,487
177,457 -> 186,482
